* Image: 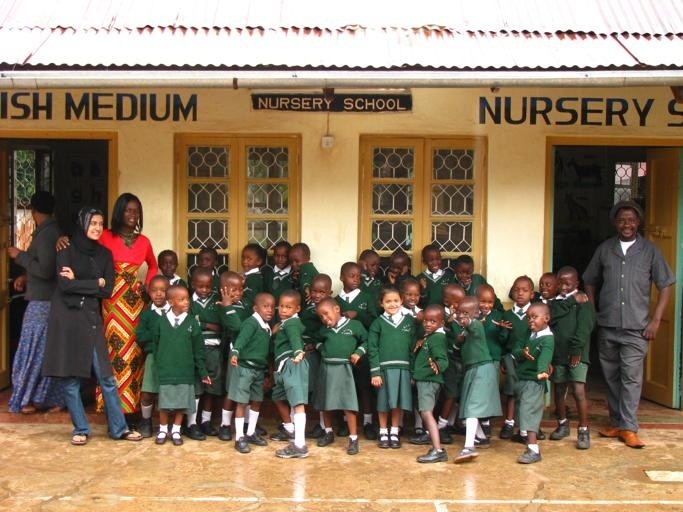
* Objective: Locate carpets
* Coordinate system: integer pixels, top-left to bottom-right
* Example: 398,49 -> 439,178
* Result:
0,377 -> 681,428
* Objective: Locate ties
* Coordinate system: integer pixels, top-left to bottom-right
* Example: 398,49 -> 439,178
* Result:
157,269 -> 568,342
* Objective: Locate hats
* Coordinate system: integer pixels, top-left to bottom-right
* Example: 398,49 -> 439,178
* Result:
608,199 -> 644,228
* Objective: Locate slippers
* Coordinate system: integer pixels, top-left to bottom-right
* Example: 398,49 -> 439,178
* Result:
120,430 -> 143,440
71,435 -> 87,446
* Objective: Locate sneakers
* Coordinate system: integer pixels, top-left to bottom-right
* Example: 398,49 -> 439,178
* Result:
454,447 -> 479,464
275,442 -> 308,458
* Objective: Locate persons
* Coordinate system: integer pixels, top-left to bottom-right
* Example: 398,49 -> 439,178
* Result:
580,198 -> 677,446
137,242 -> 370,458
332,245 -> 511,464
41,205 -> 143,445
54,193 -> 158,436
8,190 -> 61,413
499,266 -> 594,463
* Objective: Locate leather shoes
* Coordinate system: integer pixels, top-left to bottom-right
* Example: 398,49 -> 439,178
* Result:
20,404 -> 36,413
48,406 -> 64,413
347,436 -> 358,455
575,425 -> 591,451
549,418 -> 571,442
234,437 -> 250,453
616,429 -> 646,449
415,448 -> 448,463
516,447 -> 541,464
128,415 -> 548,450
598,427 -> 619,438
